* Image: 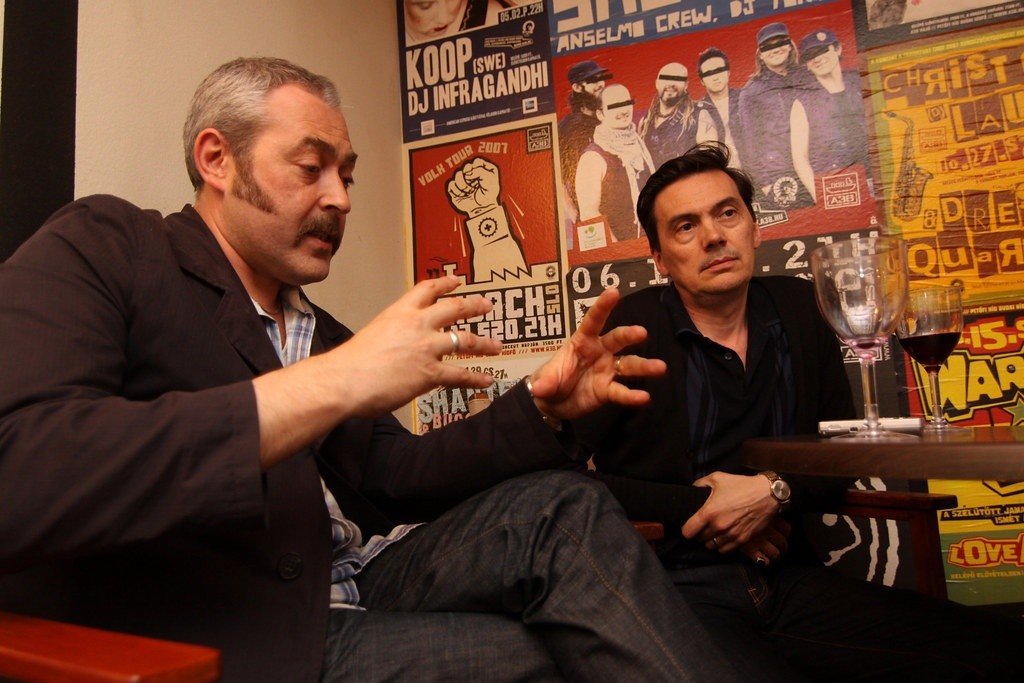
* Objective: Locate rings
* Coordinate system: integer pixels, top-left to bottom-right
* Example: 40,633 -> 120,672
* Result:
613,354 -> 625,378
712,537 -> 721,548
446,331 -> 458,353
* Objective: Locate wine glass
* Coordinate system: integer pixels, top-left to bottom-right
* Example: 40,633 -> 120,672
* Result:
894,285 -> 970,432
811,237 -> 920,441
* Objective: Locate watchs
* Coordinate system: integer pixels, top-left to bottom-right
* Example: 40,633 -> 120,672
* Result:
757,468 -> 792,522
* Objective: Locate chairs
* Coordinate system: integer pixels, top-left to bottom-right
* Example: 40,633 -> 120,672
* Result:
630,483 -> 958,683
0,610 -> 221,683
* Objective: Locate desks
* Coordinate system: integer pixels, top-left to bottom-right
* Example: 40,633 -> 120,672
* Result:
741,420 -> 1024,486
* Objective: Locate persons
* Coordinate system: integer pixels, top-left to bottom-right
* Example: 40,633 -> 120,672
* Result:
0,53 -> 742,683
557,21 -> 873,243
588,139 -> 1024,683
